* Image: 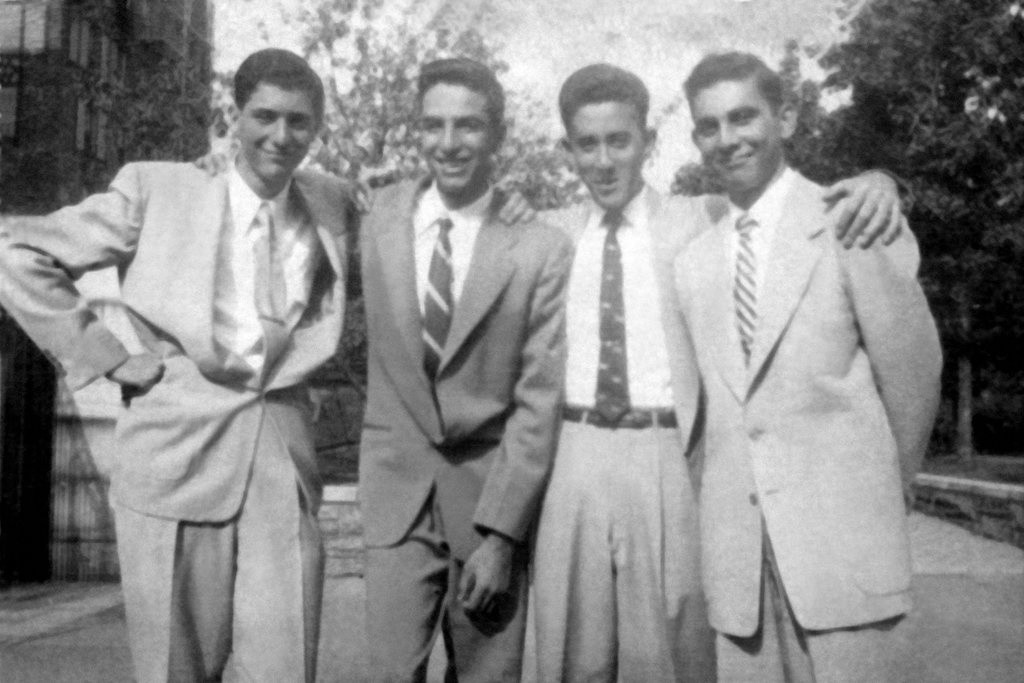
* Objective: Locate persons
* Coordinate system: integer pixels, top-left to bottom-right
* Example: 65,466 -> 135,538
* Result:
343,64 -> 911,683
675,50 -> 947,683
0,47 -> 534,683
194,57 -> 572,683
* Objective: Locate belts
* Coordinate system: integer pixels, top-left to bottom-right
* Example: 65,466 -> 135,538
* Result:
563,405 -> 677,429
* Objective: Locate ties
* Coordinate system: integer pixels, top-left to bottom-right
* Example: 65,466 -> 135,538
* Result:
423,218 -> 455,380
733,214 -> 757,365
595,210 -> 631,424
252,207 -> 289,385
